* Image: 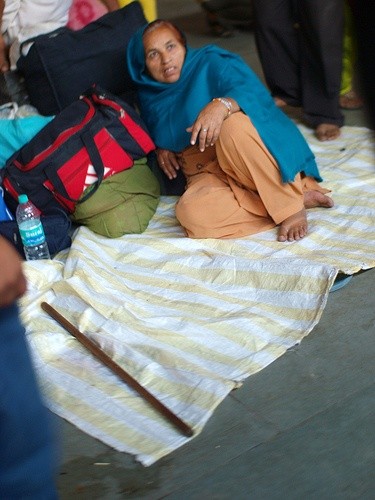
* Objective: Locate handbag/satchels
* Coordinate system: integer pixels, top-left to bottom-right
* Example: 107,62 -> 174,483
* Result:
0,82 -> 162,238
14,1 -> 151,117
0,202 -> 74,260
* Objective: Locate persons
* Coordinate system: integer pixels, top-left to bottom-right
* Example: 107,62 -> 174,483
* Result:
0,0 -> 375,142
0,188 -> 58,500
126,19 -> 333,242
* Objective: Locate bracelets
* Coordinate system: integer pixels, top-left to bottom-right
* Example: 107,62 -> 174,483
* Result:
213,96 -> 232,116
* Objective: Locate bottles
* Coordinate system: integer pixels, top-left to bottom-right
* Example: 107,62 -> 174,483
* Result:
16,194 -> 51,261
